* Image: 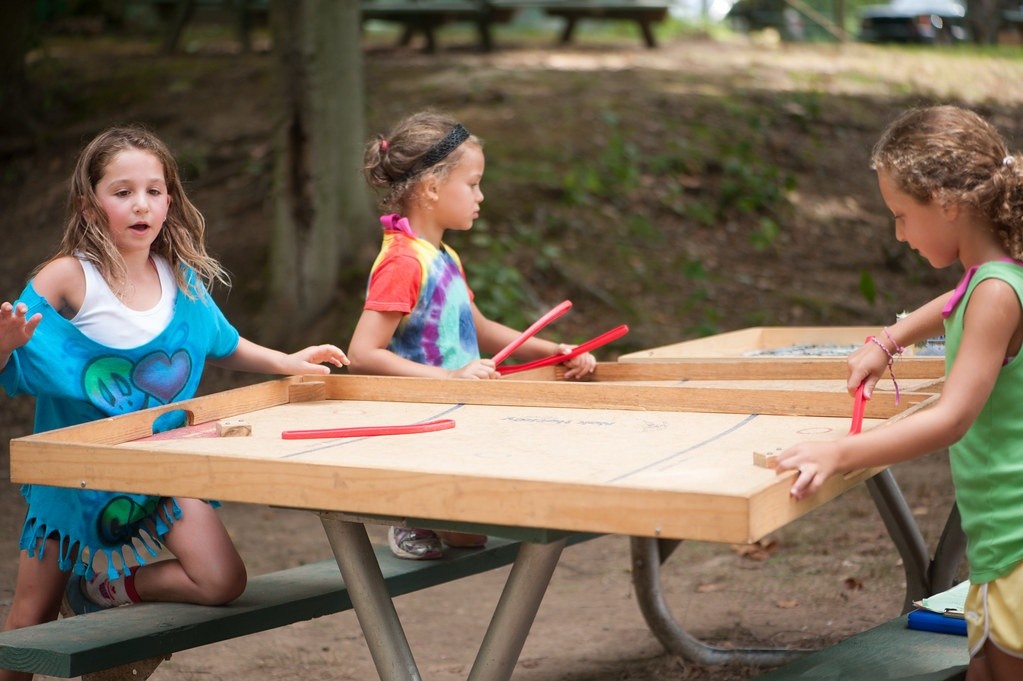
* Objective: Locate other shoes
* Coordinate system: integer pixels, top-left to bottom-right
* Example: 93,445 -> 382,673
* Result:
59,574 -> 104,619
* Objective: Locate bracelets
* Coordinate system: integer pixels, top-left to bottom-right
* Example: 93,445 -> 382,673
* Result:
871,327 -> 906,407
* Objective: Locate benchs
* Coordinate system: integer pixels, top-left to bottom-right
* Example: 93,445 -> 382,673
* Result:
742,573 -> 974,680
0,531 -> 611,681
538,4 -> 667,50
366,6 -> 517,53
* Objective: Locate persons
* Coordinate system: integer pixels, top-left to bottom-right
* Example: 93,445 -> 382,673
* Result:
763,105 -> 1023,678
1,123 -> 349,681
345,107 -> 598,563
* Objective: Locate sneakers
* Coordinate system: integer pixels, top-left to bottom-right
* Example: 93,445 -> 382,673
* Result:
387,526 -> 444,559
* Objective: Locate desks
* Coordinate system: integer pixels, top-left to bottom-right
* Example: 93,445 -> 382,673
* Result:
10,322 -> 965,680
